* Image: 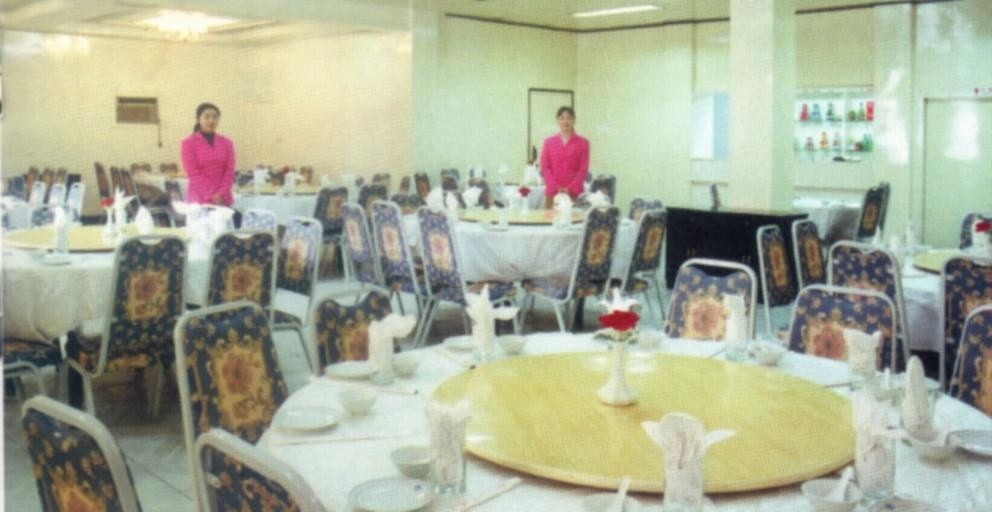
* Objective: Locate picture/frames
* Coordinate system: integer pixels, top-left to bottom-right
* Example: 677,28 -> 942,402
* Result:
527,88 -> 574,167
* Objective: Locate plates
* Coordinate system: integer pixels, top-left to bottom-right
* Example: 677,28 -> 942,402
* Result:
269,313 -> 991,512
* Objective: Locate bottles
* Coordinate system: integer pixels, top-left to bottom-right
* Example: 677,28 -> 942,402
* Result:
795,99 -> 873,152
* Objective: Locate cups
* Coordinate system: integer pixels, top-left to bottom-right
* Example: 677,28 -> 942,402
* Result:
426,197 -> 573,228
3,209 -> 237,264
886,227 -> 992,277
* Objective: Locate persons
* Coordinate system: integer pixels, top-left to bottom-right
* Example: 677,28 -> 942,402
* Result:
181,103 -> 235,207
540,107 -> 590,209
